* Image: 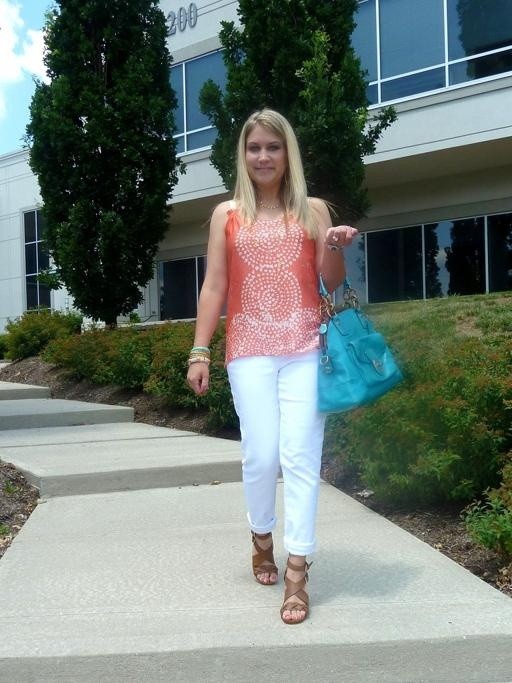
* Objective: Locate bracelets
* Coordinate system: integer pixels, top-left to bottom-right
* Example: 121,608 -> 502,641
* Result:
187,346 -> 212,368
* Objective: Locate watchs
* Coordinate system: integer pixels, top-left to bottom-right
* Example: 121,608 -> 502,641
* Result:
323,237 -> 345,253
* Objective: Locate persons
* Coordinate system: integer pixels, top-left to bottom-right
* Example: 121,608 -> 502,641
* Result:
186,105 -> 361,625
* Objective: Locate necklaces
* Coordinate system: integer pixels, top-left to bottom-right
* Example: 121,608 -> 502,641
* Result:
255,195 -> 283,210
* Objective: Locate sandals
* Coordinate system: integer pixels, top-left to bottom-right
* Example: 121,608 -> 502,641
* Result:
251,530 -> 278,585
280,557 -> 314,625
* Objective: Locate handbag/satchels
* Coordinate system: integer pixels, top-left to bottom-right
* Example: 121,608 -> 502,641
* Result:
313,272 -> 403,415
